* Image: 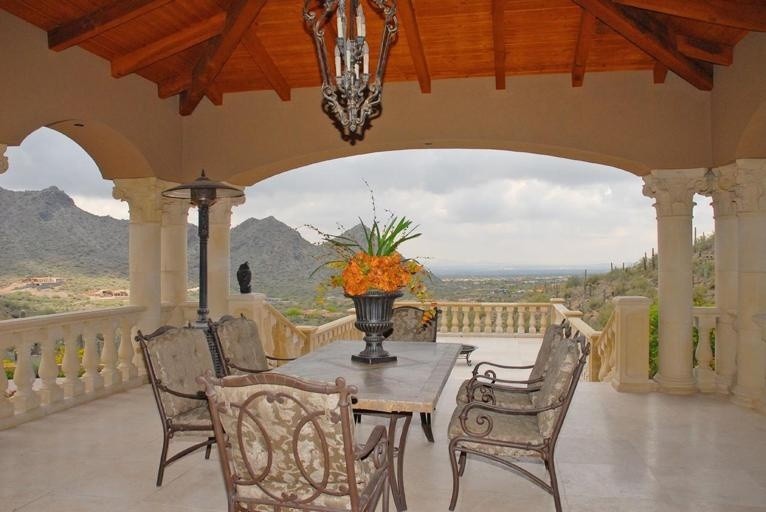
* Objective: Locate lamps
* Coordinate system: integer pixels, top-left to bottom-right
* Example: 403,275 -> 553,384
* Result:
303,0 -> 398,133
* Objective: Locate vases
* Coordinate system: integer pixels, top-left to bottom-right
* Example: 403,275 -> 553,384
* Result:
343,292 -> 402,364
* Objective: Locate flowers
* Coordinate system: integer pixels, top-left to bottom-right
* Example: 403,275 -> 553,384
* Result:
284,179 -> 441,332
